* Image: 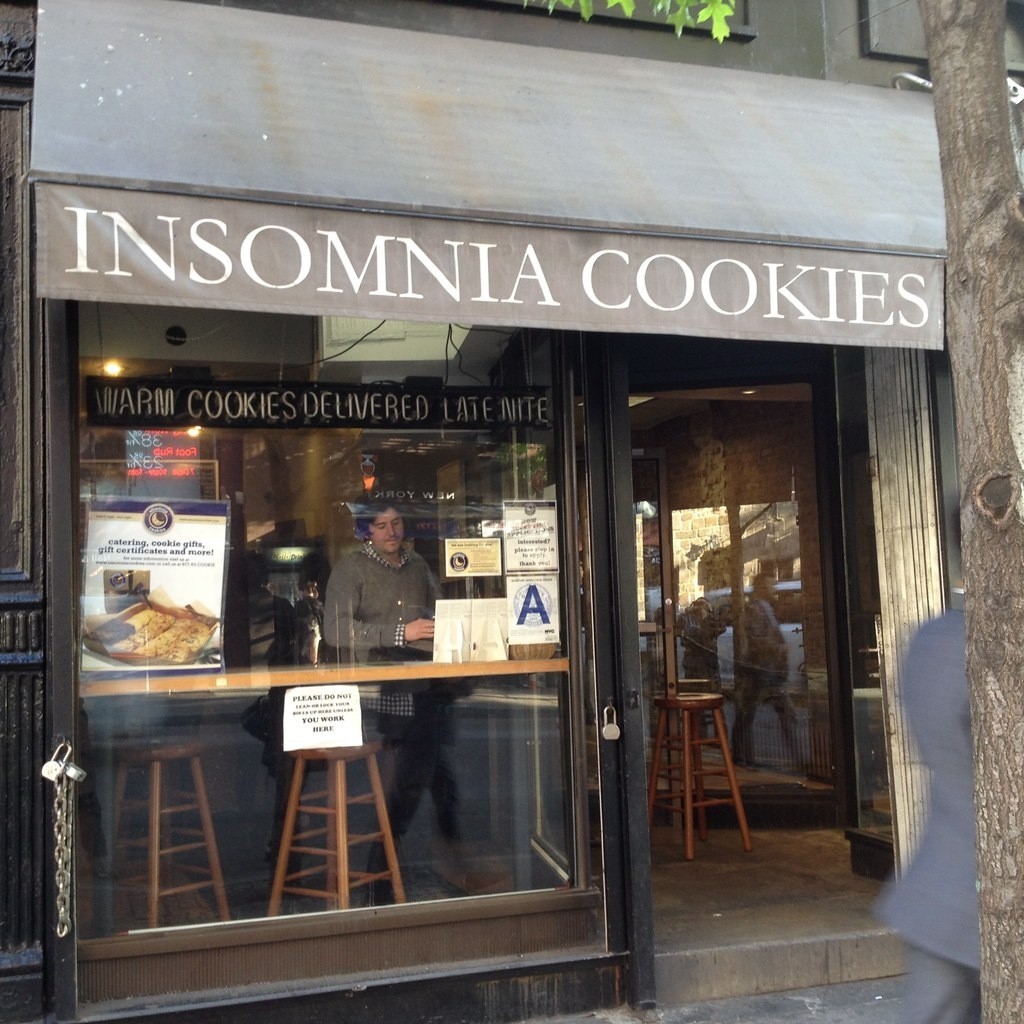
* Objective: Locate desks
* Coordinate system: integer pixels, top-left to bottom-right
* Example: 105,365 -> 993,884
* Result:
75,655 -> 570,700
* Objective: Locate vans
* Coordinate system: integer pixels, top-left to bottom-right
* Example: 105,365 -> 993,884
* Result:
677,581 -> 803,683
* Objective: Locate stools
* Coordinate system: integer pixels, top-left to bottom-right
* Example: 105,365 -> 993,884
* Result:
265,743 -> 408,918
107,739 -> 234,928
647,691 -> 754,861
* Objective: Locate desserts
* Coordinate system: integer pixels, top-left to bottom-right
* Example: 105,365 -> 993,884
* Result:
92,613 -> 209,662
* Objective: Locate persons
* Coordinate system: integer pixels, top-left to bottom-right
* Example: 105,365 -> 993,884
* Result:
367,678 -> 484,907
324,494 -> 446,891
879,505 -> 985,1024
241,686 -> 328,895
729,571 -> 830,781
224,549 -> 335,664
673,597 -> 727,749
